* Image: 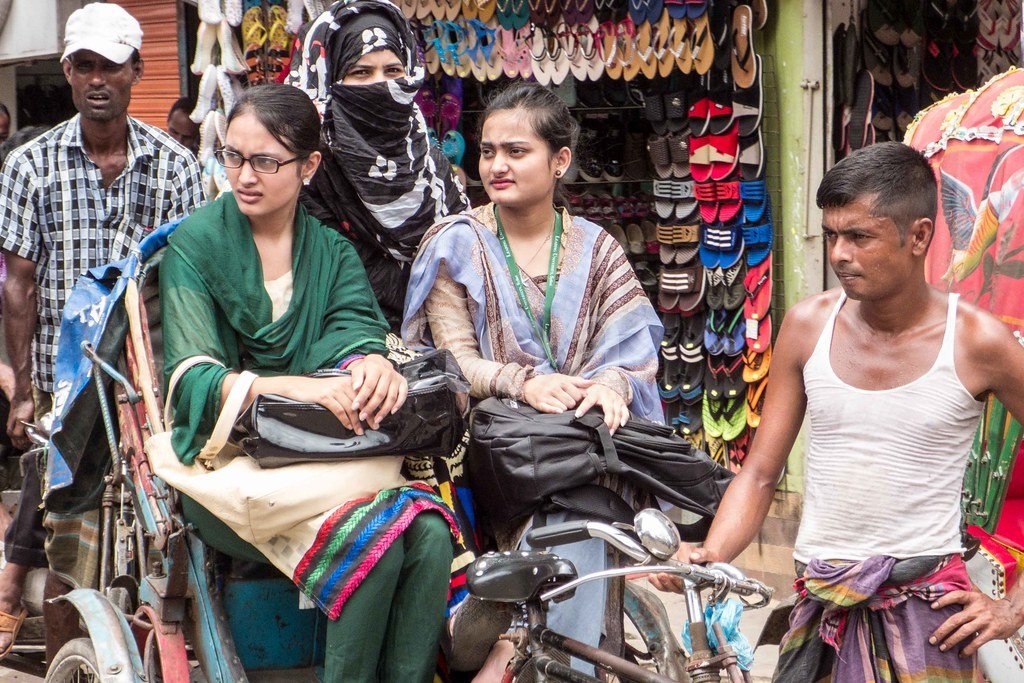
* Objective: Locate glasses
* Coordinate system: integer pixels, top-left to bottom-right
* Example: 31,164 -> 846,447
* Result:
213,147 -> 308,174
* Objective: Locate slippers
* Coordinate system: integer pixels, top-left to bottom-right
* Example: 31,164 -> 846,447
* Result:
193,0 -> 775,467
833,2 -> 1024,159
0,607 -> 28,660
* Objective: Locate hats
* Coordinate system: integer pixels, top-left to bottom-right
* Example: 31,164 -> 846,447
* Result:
60,2 -> 142,66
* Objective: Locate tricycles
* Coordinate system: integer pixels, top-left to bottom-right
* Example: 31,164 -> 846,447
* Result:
43,214 -> 776,683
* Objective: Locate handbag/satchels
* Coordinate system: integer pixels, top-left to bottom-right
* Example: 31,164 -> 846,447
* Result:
233,348 -> 470,468
463,398 -> 736,542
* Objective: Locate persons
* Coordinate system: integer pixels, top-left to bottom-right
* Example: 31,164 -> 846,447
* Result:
166,97 -> 198,154
160,81 -> 454,683
0,125 -> 51,171
0,103 -> 11,146
647,142 -> 1024,683
0,0 -> 208,660
300,0 -> 470,334
400,88 -> 666,683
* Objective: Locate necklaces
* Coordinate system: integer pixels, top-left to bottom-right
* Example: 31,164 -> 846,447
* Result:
519,219 -> 553,287
